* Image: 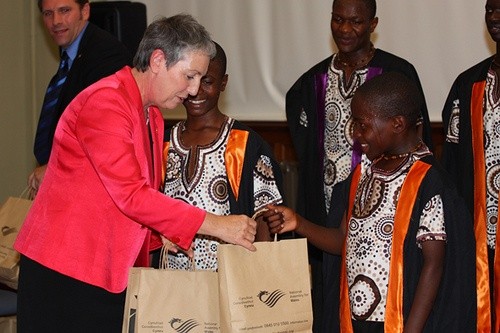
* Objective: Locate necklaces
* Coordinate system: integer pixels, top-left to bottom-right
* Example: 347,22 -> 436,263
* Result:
337,41 -> 374,66
383,137 -> 423,160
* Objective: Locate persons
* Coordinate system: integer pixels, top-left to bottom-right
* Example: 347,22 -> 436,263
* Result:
27,0 -> 128,188
284,0 -> 434,333
262,72 -> 446,333
441,0 -> 500,333
144,41 -> 284,271
15,13 -> 258,333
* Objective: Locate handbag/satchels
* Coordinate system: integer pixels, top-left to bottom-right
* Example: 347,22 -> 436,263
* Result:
0,185 -> 39,289
121,242 -> 219,333
218,208 -> 313,333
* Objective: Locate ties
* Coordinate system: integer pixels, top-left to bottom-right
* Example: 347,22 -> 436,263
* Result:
33,51 -> 70,166
